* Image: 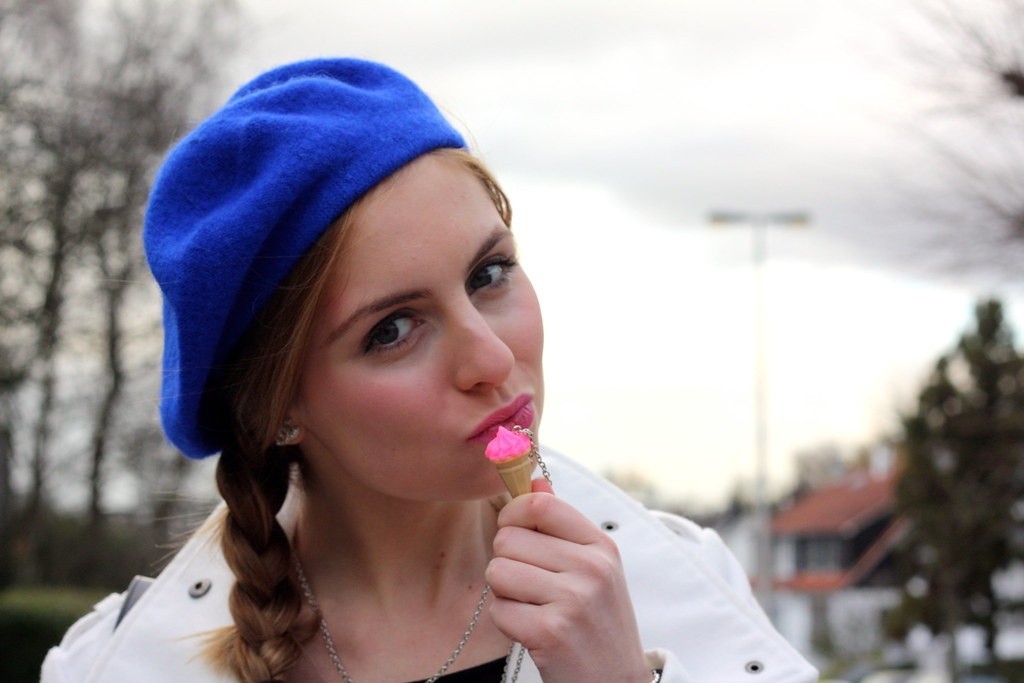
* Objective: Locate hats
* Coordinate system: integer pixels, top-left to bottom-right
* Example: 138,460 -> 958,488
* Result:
144,58 -> 468,460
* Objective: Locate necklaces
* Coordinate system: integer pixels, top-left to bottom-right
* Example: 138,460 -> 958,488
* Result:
294,425 -> 553,682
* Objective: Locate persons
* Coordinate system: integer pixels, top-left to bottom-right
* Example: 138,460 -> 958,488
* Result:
39,57 -> 821,682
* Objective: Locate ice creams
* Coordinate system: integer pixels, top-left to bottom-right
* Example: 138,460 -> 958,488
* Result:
485,425 -> 532,498
